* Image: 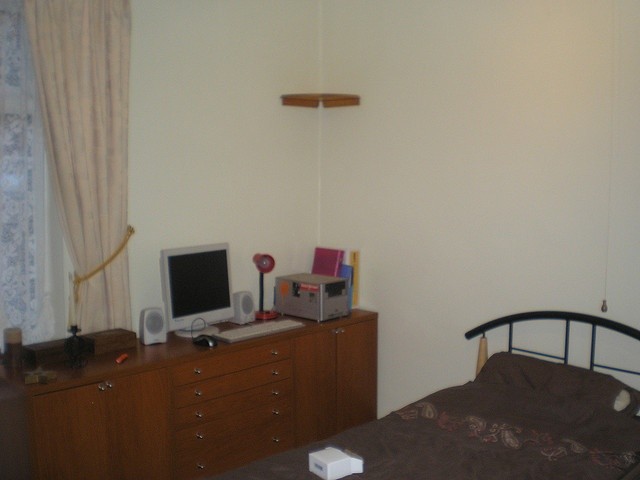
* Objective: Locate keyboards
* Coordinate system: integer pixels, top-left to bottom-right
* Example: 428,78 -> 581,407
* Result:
215,318 -> 306,343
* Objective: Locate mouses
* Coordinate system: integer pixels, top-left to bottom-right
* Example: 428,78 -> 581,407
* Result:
193,335 -> 217,347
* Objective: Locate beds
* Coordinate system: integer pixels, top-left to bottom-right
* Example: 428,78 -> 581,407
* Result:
210,310 -> 640,479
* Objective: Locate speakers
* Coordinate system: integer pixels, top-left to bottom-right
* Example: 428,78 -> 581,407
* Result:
139,308 -> 167,344
228,291 -> 256,324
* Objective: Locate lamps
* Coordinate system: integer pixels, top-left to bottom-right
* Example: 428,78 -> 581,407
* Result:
252,253 -> 277,319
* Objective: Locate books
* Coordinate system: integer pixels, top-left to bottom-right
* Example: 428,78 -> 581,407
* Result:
311,247 -> 360,316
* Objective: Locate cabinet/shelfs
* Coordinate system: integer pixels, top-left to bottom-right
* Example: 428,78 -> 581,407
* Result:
167,341 -> 297,480
292,322 -> 378,448
174,368 -> 476,480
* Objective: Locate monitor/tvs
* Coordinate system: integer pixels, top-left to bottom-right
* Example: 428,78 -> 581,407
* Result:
160,242 -> 235,339
